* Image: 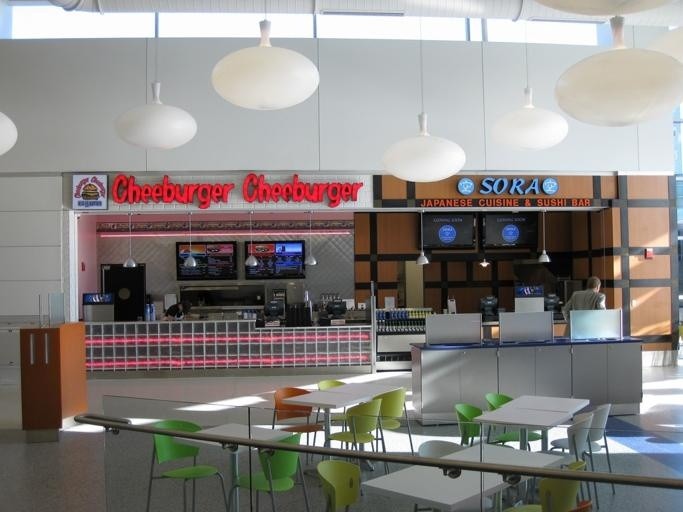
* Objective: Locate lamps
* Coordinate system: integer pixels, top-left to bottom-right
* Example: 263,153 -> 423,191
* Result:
1,105 -> 23,167
549,16 -> 683,131
114,36 -> 200,153
479,210 -> 491,269
244,208 -> 259,267
537,208 -> 551,263
538,0 -> 676,20
493,71 -> 569,152
121,210 -> 138,268
183,212 -> 197,268
414,208 -> 430,264
383,94 -> 467,185
303,209 -> 318,265
208,16 -> 324,117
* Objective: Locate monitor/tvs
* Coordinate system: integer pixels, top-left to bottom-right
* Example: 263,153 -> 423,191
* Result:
264,303 -> 284,316
328,302 -> 346,314
245,240 -> 305,280
176,241 -> 237,280
482,211 -> 538,249
418,213 -> 477,250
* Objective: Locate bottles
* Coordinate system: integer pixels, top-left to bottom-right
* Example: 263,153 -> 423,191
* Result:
303,290 -> 308,308
149,304 -> 155,321
280,244 -> 285,254
318,293 -> 339,311
376,308 -> 432,333
312,304 -> 318,320
242,304 -> 256,320
145,304 -> 150,321
258,256 -> 302,275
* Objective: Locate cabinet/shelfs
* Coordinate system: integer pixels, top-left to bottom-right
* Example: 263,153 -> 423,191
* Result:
21,323 -> 87,431
498,343 -> 571,418
572,337 -> 643,407
410,337 -> 495,427
0,315 -> 45,430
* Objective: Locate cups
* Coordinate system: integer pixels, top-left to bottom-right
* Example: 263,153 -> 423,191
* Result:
442,309 -> 447,314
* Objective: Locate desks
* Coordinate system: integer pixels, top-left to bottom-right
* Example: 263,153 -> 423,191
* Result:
191,420 -> 295,512
279,382 -> 406,450
357,441 -> 566,511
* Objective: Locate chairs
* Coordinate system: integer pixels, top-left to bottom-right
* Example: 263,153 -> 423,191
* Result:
372,385 -> 414,477
315,459 -> 362,511
227,430 -> 310,511
418,439 -> 460,461
271,386 -> 322,474
313,379 -> 354,464
452,391 -> 616,512
326,397 -> 382,497
146,419 -> 231,511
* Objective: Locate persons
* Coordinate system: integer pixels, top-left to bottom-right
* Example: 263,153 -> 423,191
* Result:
560,276 -> 606,336
161,302 -> 183,321
